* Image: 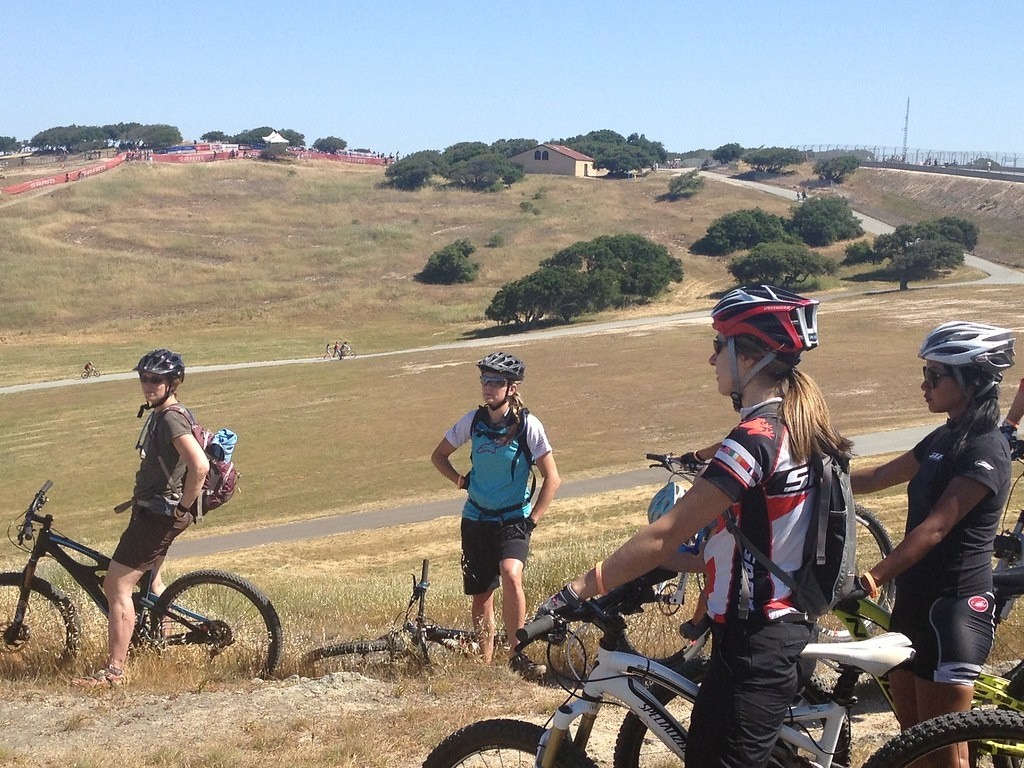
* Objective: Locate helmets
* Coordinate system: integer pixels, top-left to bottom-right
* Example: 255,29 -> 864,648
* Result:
476,352 -> 525,380
648,483 -> 700,553
710,284 -> 819,353
133,348 -> 185,382
918,321 -> 1017,375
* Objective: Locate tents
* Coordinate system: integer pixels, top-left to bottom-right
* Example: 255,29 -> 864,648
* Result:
262,131 -> 289,144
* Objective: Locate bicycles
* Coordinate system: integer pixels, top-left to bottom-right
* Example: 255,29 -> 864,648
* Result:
309,558 -> 510,678
1,479 -> 283,683
618,452 -> 895,674
973,433 -> 1024,700
423,565 -> 1024,768
337,347 -> 356,360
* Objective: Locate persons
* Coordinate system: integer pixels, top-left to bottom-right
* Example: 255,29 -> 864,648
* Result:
431,353 -> 562,680
680,440 -> 724,639
534,284 -> 853,768
85,361 -> 93,378
340,342 -> 349,359
230,149 -> 247,159
71,349 -> 211,687
213,151 -> 216,160
66,172 -> 68,182
849,321 -> 1017,768
324,344 -> 331,359
333,341 -> 340,357
797,188 -> 806,201
883,155 -> 958,166
998,378 -> 1024,441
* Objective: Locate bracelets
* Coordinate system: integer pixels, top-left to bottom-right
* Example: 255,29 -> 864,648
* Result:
177,502 -> 189,512
693,451 -> 705,463
862,572 -> 877,599
596,561 -> 608,596
458,475 -> 463,489
1005,417 -> 1020,428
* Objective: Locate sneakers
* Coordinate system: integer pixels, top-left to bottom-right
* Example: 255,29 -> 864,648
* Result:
509,653 -> 547,680
71,664 -> 132,687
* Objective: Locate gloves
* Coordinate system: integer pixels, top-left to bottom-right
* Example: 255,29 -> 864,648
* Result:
844,575 -> 869,599
524,517 -> 537,532
1000,419 -> 1018,442
462,471 -> 471,490
680,450 -> 704,471
533,584 -> 582,645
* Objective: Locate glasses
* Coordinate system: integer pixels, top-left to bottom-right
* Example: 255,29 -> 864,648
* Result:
478,375 -> 508,386
712,340 -> 746,352
922,364 -> 964,389
139,373 -> 168,383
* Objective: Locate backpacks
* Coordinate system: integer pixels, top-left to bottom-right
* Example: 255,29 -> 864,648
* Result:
150,405 -> 239,517
726,402 -> 857,617
